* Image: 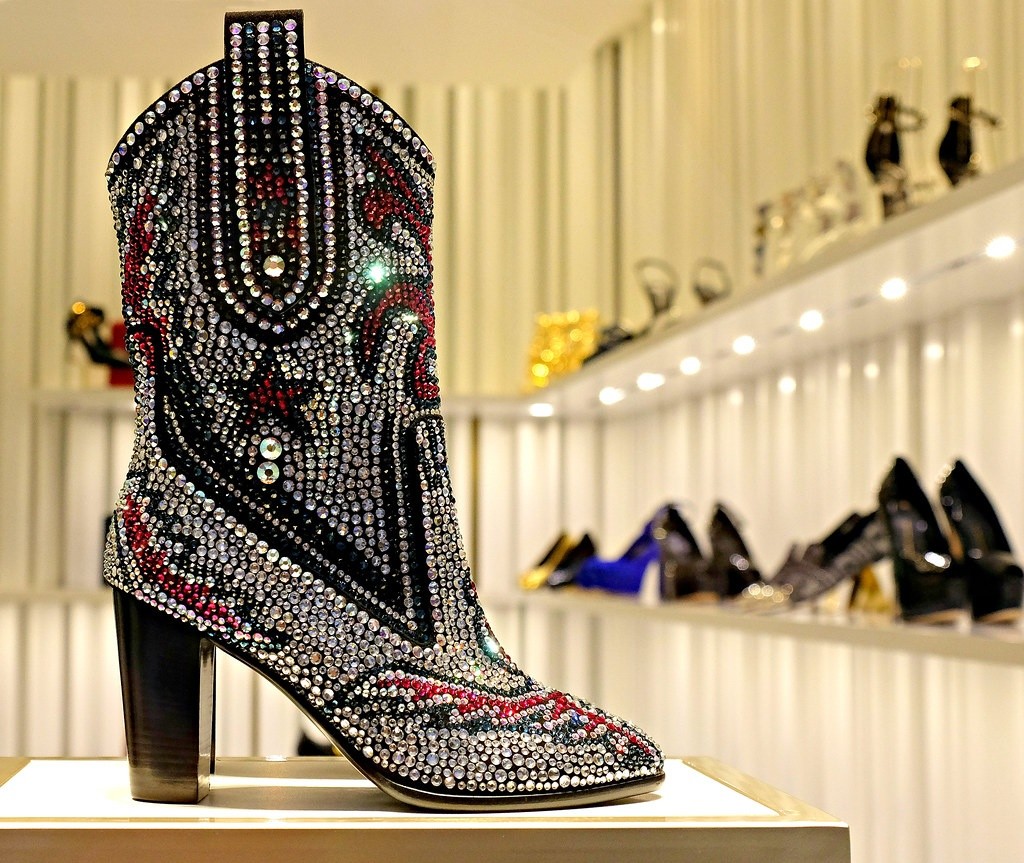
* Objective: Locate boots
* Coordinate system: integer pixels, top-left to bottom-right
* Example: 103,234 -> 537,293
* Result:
102,11 -> 665,812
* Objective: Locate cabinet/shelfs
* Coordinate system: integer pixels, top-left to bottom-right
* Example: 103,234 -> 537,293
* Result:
35,149 -> 1024,672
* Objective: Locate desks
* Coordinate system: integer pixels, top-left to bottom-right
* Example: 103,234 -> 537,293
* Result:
0,756 -> 850,863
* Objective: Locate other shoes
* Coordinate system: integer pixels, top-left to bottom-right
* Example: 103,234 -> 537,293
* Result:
66,306 -> 131,370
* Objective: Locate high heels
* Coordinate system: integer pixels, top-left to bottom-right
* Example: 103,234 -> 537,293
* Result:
582,94 -> 1005,368
517,458 -> 1024,626
